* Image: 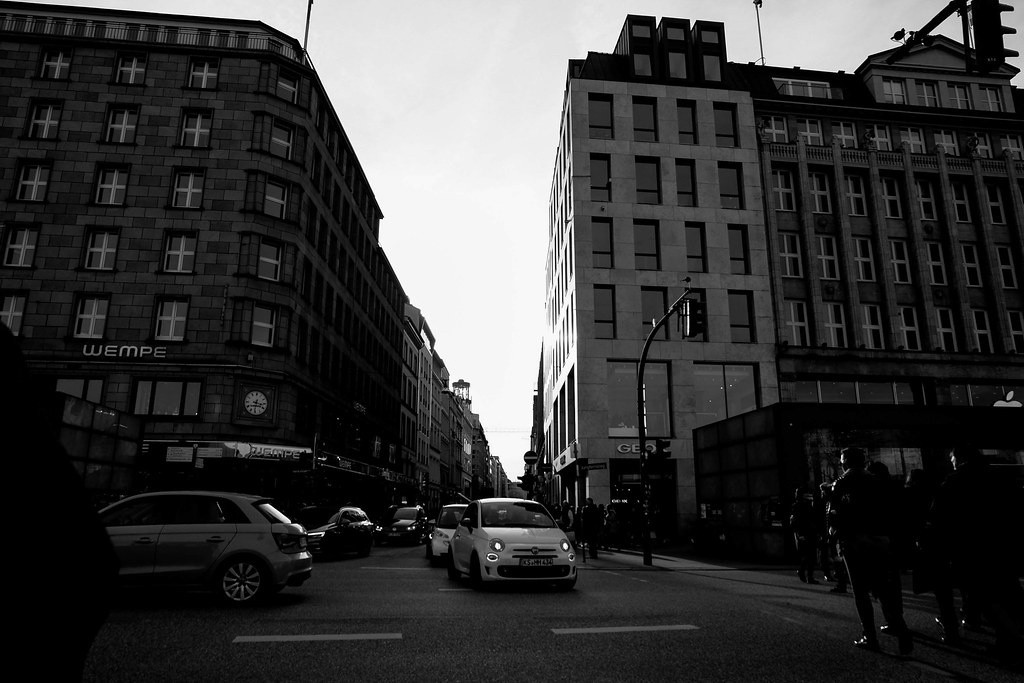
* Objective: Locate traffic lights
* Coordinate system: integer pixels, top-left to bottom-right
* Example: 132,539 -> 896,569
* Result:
654,439 -> 672,459
517,474 -> 530,492
681,297 -> 705,340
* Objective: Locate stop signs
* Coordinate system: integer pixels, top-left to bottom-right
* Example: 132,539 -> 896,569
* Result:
523,451 -> 538,466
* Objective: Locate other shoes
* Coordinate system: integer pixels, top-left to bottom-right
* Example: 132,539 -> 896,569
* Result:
879,624 -> 901,637
809,579 -> 821,584
935,616 -> 959,629
797,571 -> 807,582
852,638 -> 880,650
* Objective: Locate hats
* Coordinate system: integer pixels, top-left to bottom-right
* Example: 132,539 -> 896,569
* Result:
840,447 -> 865,467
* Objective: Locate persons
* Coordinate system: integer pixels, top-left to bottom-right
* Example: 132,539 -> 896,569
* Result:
788,446 -> 1023,658
545,498 -> 617,559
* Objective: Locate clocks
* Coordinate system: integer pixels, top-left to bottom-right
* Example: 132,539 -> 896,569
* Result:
243,389 -> 268,416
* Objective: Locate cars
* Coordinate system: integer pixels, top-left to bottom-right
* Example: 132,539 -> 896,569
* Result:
427,504 -> 491,562
446,498 -> 577,592
291,506 -> 375,561
97,489 -> 314,611
373,504 -> 428,547
